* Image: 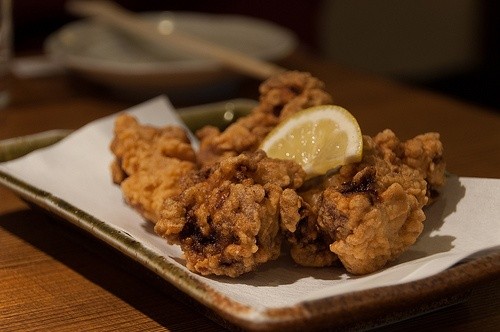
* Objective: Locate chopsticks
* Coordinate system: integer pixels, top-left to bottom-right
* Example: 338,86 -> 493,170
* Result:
72,1 -> 292,82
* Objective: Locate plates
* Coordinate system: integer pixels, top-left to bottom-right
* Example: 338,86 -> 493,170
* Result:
0,98 -> 499,332
49,14 -> 297,93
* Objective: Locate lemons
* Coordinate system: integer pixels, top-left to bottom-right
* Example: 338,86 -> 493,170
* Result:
256,104 -> 363,179
